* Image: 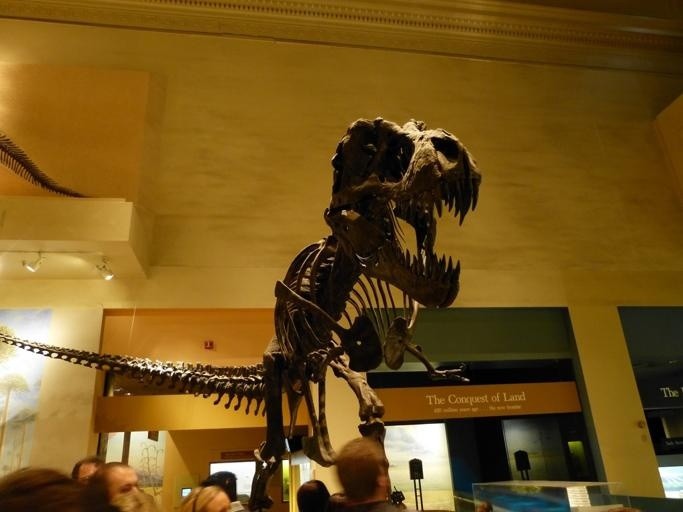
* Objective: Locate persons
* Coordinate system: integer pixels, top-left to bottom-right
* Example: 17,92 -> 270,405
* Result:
179,484 -> 231,512
89,461 -> 140,503
296,479 -> 331,511
327,436 -> 406,512
0,466 -> 121,512
108,488 -> 159,512
69,455 -> 105,486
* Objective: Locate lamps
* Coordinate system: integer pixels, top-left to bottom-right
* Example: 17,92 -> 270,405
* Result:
0,250 -> 114,280
